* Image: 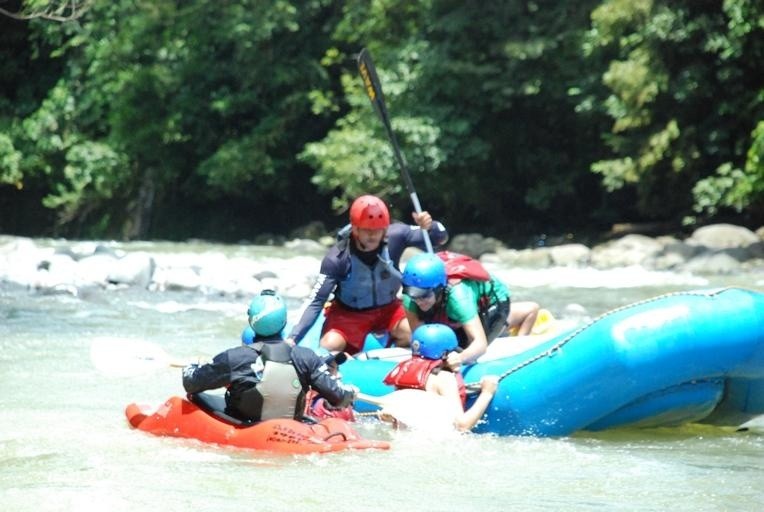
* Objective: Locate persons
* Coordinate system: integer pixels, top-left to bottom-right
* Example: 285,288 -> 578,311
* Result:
401,251 -> 511,372
311,347 -> 383,419
499,301 -> 539,338
242,326 -> 285,346
182,287 -> 360,423
384,323 -> 498,432
285,195 -> 449,349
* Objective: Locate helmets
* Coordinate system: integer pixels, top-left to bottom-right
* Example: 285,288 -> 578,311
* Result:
413,323 -> 457,360
402,254 -> 447,298
350,194 -> 390,230
242,293 -> 287,345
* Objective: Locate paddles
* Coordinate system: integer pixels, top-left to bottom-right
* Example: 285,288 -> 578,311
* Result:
358,49 -> 432,253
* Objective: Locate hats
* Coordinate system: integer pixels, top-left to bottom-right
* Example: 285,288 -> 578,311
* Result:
311,348 -> 346,363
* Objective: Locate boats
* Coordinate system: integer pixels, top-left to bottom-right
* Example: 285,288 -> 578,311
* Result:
121,389 -> 403,453
238,279 -> 763,445
